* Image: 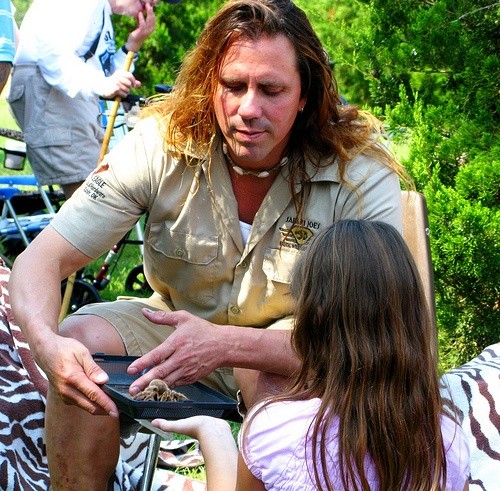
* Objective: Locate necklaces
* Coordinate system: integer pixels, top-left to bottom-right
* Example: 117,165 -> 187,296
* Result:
222,137 -> 290,178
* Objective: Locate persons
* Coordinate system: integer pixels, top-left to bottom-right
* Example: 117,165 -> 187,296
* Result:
5,0 -> 158,210
10,0 -> 402,491
0,0 -> 20,96
150,219 -> 470,491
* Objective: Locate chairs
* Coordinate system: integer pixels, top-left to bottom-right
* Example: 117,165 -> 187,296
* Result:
0,175 -> 158,314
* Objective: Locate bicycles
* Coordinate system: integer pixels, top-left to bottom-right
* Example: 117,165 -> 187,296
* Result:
0,84 -> 171,315
